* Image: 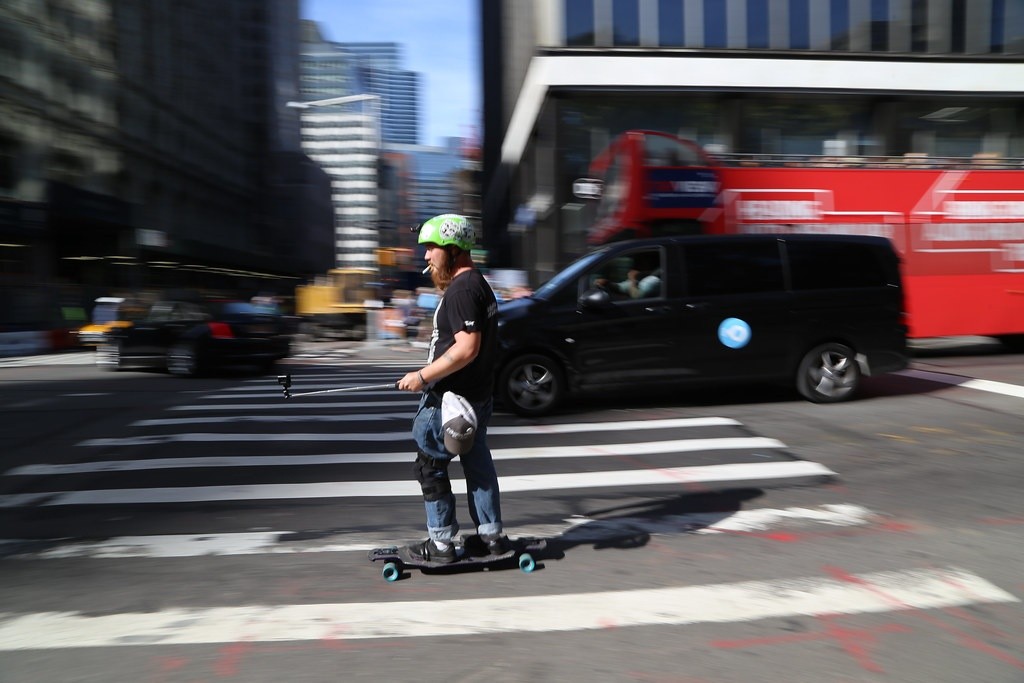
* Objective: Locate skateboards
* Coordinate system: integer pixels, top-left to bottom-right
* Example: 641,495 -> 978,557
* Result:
368,535 -> 549,583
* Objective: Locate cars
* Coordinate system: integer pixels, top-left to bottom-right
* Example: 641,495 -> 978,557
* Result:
77,297 -> 130,344
101,293 -> 291,380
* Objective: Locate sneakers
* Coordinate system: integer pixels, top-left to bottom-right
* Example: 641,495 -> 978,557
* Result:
407,538 -> 459,563
461,534 -> 511,555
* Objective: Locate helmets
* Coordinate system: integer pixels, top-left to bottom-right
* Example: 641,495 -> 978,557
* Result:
417,214 -> 476,250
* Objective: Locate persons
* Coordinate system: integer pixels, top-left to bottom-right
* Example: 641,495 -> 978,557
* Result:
399,213 -> 509,561
594,253 -> 661,299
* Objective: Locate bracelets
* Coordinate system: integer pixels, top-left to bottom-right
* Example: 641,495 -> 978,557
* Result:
418,370 -> 429,385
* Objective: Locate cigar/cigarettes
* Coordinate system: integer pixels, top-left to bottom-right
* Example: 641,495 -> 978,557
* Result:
423,264 -> 432,274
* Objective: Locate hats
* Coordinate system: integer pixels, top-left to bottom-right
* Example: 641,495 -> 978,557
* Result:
441,391 -> 478,455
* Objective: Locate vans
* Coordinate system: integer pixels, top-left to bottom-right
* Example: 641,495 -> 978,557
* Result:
488,233 -> 912,420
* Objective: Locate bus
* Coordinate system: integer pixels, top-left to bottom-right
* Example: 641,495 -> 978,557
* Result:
574,126 -> 1024,349
